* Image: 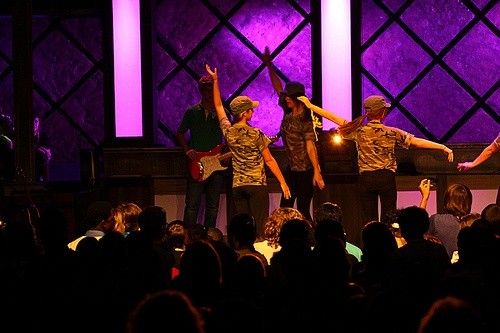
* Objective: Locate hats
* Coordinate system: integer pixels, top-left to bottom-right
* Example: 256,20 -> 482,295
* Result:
198,76 -> 214,86
228,96 -> 259,118
280,81 -> 304,95
363,96 -> 391,112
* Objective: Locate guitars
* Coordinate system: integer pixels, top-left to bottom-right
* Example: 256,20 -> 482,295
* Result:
186,136 -> 279,183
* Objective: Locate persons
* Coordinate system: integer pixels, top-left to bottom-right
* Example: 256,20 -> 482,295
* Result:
0,111 -> 500,332
297,94 -> 455,225
177,74 -> 228,229
205,64 -> 293,238
263,48 -> 324,228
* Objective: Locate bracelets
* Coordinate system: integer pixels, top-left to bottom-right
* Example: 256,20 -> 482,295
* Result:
443,147 -> 447,151
